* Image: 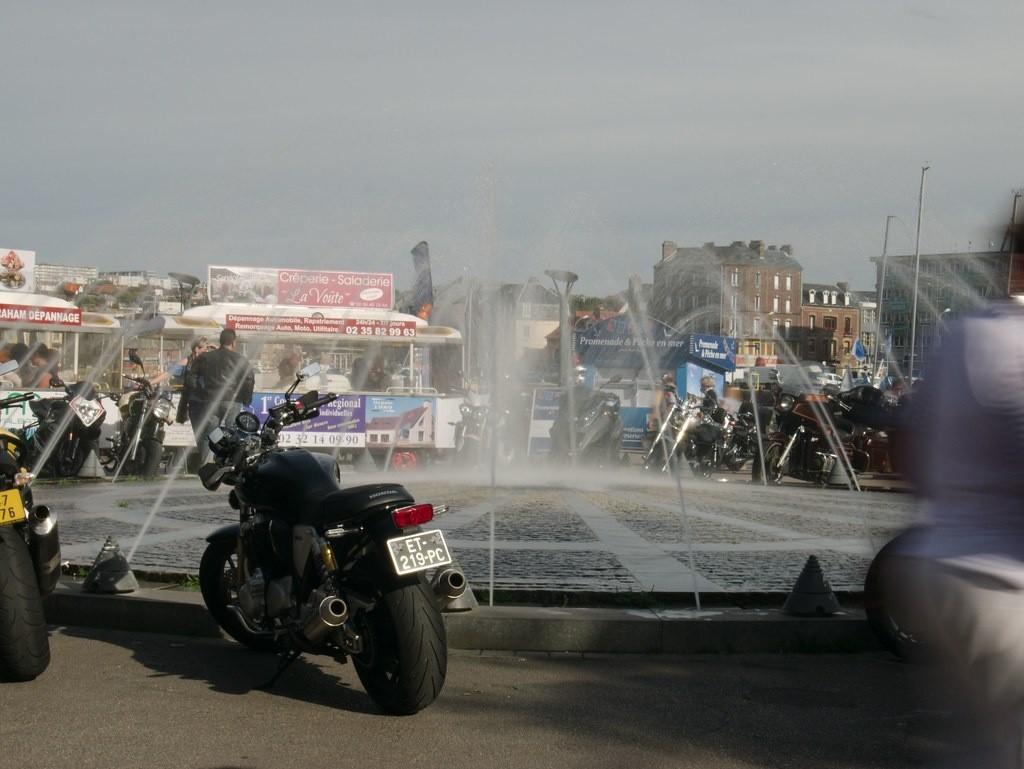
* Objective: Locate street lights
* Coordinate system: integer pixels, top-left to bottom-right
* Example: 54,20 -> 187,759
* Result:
869,215 -> 898,387
544,269 -> 578,472
906,164 -> 936,390
937,308 -> 950,358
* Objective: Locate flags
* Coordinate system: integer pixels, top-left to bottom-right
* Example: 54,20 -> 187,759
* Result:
883,328 -> 893,360
852,339 -> 866,362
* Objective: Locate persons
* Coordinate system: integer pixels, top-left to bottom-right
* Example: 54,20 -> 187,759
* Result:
272,353 -> 299,391
350,354 -> 392,390
147,337 -> 219,457
699,376 -> 717,411
0,343 -> 58,391
187,329 -> 255,468
661,371 -> 676,388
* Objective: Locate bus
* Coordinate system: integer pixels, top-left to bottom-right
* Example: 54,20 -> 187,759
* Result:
448,351 -> 536,475
0,289 -> 462,473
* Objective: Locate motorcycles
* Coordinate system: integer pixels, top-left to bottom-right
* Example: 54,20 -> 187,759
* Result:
545,372 -> 626,474
197,358 -> 469,714
104,349 -> 194,480
0,360 -> 63,683
638,361 -> 930,499
25,371 -> 121,480
